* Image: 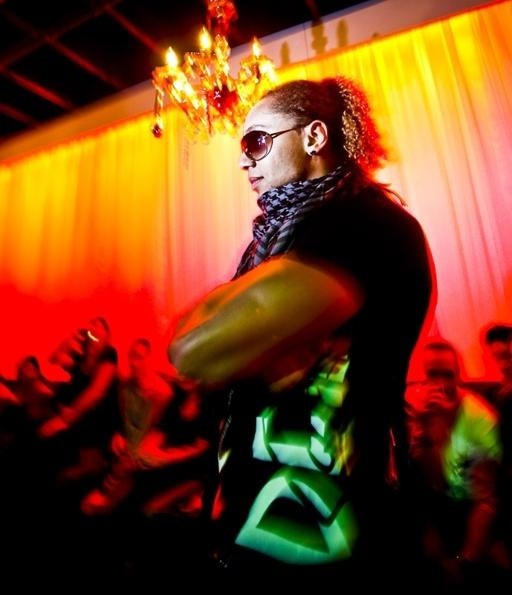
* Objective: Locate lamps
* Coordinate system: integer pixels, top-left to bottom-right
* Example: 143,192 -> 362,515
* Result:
150,0 -> 278,141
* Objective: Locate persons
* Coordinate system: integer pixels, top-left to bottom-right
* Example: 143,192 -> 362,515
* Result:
165,74 -> 437,594
1,319 -> 215,595
406,325 -> 511,595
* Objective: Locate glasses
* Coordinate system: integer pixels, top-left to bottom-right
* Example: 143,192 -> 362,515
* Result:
241,123 -> 311,161
